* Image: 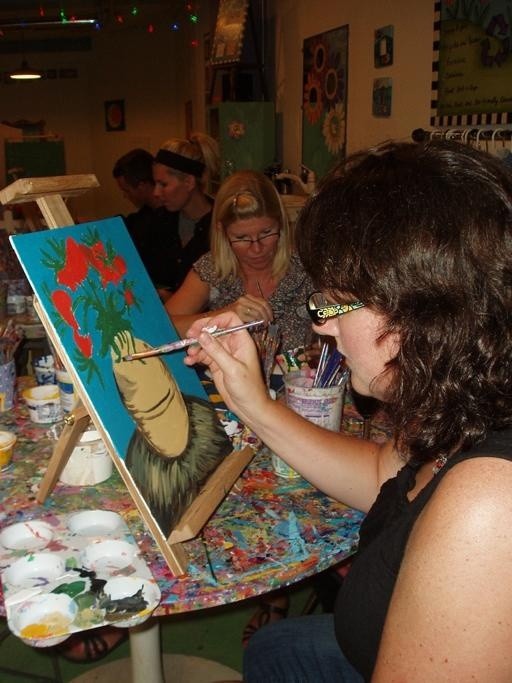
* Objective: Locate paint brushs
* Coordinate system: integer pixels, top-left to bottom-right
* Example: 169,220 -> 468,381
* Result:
0,308 -> 25,365
313,341 -> 348,388
118,317 -> 270,361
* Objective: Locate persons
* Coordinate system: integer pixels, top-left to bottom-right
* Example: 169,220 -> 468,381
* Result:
162,171 -> 321,355
190,138 -> 512,683
112,149 -> 162,228
139,134 -> 215,294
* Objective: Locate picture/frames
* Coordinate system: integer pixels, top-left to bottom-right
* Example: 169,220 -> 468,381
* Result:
301,24 -> 348,182
371,82 -> 392,119
430,9 -> 511,127
374,24 -> 393,71
104,98 -> 126,132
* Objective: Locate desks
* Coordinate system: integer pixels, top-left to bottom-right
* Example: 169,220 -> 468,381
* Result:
0,374 -> 369,682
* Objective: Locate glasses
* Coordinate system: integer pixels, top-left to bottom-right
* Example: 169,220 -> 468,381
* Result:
225,232 -> 283,250
303,288 -> 368,328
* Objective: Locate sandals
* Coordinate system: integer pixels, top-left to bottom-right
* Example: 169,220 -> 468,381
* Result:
241,597 -> 291,646
61,626 -> 129,665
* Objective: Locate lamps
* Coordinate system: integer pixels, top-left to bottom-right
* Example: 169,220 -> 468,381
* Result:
8,32 -> 42,80
